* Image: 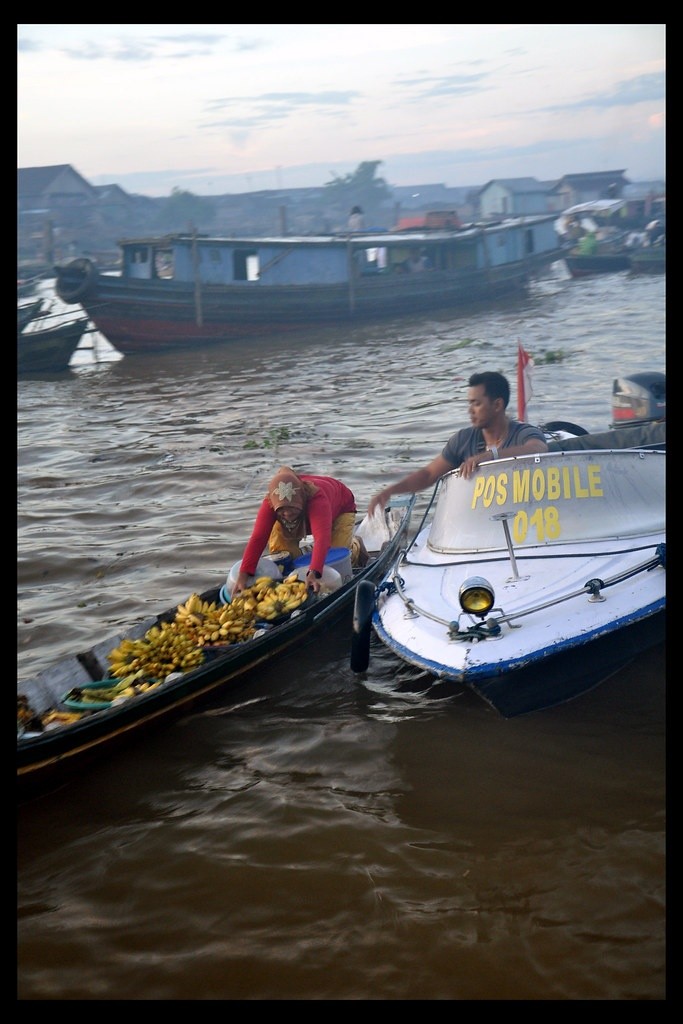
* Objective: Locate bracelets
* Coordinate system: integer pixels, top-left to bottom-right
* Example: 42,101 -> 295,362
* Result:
309,569 -> 316,574
491,447 -> 499,460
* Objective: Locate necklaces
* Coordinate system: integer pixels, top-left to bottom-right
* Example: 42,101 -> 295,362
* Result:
485,432 -> 506,452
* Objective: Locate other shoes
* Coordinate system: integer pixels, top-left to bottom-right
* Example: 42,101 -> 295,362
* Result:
355,536 -> 369,567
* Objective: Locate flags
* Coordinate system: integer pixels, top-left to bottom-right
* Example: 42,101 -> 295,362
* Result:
517,344 -> 536,424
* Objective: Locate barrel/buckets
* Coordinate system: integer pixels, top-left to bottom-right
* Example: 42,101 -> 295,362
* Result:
262,550 -> 295,578
292,546 -> 353,583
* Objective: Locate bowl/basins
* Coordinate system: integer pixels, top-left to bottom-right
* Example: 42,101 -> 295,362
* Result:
242,578 -> 316,626
60,677 -> 160,711
285,565 -> 343,595
218,556 -> 284,607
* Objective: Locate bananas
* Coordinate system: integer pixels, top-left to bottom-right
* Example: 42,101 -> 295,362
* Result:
106,574 -> 309,678
111,679 -> 161,700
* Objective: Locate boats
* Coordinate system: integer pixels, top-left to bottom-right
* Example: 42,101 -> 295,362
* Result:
18,498 -> 412,790
347,420 -> 668,716
52,205 -> 573,354
16,272 -> 110,373
558,198 -> 666,269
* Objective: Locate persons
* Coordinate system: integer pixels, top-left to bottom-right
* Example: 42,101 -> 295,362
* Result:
228,466 -> 371,594
348,205 -> 366,231
394,248 -> 435,274
368,372 -> 550,519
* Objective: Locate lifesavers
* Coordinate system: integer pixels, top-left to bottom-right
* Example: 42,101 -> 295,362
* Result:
55,257 -> 99,306
